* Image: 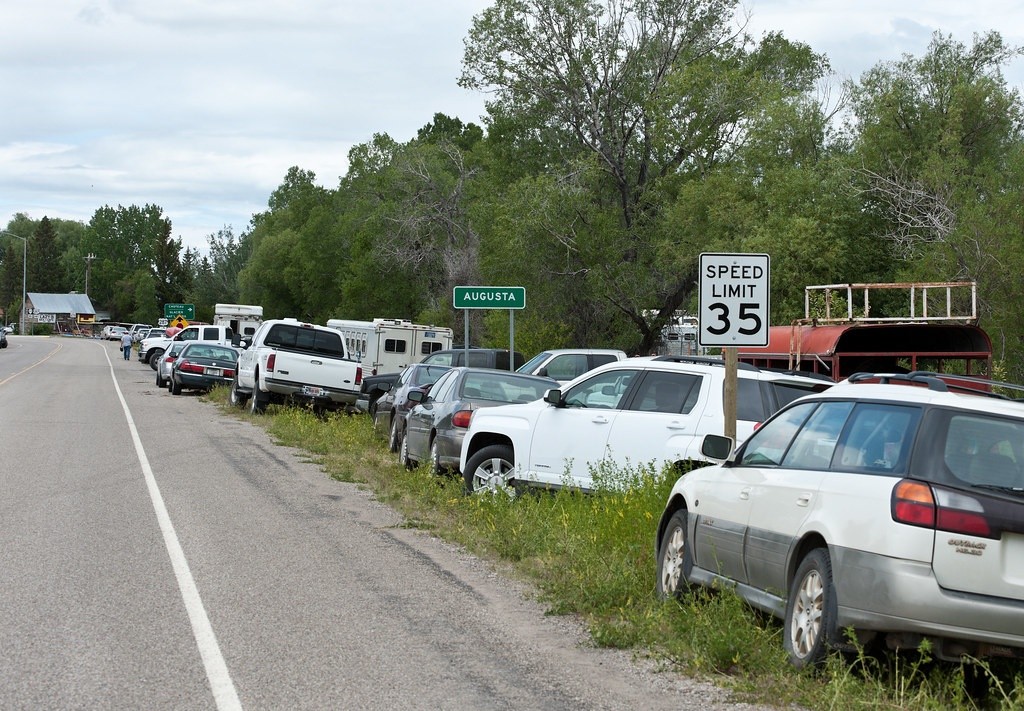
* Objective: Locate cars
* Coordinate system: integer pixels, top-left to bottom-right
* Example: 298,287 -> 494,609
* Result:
3,323 -> 16,335
110,327 -> 129,341
168,342 -> 240,395
156,342 -> 218,388
0,327 -> 8,349
399,366 -> 561,478
100,325 -> 117,338
129,324 -> 153,340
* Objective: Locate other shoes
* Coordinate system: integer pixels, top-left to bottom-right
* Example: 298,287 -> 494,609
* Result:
127,358 -> 130,360
124,358 -> 126,360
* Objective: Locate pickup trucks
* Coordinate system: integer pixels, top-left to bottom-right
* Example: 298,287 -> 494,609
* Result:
138,325 -> 245,371
466,349 -> 659,412
231,318 -> 363,420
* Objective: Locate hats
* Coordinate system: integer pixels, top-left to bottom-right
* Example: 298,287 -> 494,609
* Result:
123,330 -> 129,333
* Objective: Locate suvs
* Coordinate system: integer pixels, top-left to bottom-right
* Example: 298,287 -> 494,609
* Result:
655,372 -> 1024,673
459,353 -> 866,491
361,349 -> 526,415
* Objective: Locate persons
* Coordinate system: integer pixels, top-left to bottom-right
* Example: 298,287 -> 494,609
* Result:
121,331 -> 133,361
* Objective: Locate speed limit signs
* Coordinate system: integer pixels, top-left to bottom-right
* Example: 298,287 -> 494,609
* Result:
700,251 -> 770,350
